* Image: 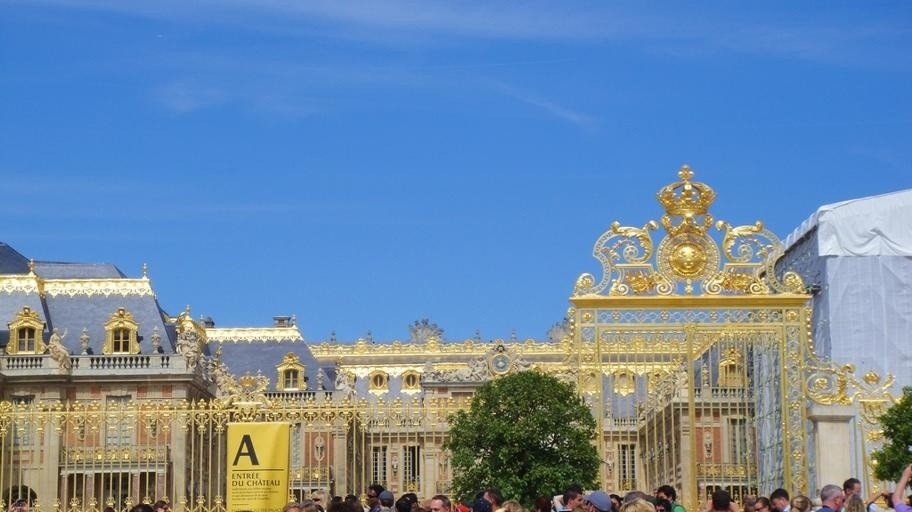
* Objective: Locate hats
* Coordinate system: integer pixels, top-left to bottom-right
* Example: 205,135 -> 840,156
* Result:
472,499 -> 490,512
380,489 -> 392,503
584,492 -> 613,511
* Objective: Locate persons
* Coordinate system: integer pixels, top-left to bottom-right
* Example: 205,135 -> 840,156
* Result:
513,355 -> 525,372
49,326 -> 73,370
104,501 -> 168,512
535,485 -> 686,512
472,356 -> 488,380
705,464 -> 912,512
8,499 -> 29,512
283,485 -> 528,512
